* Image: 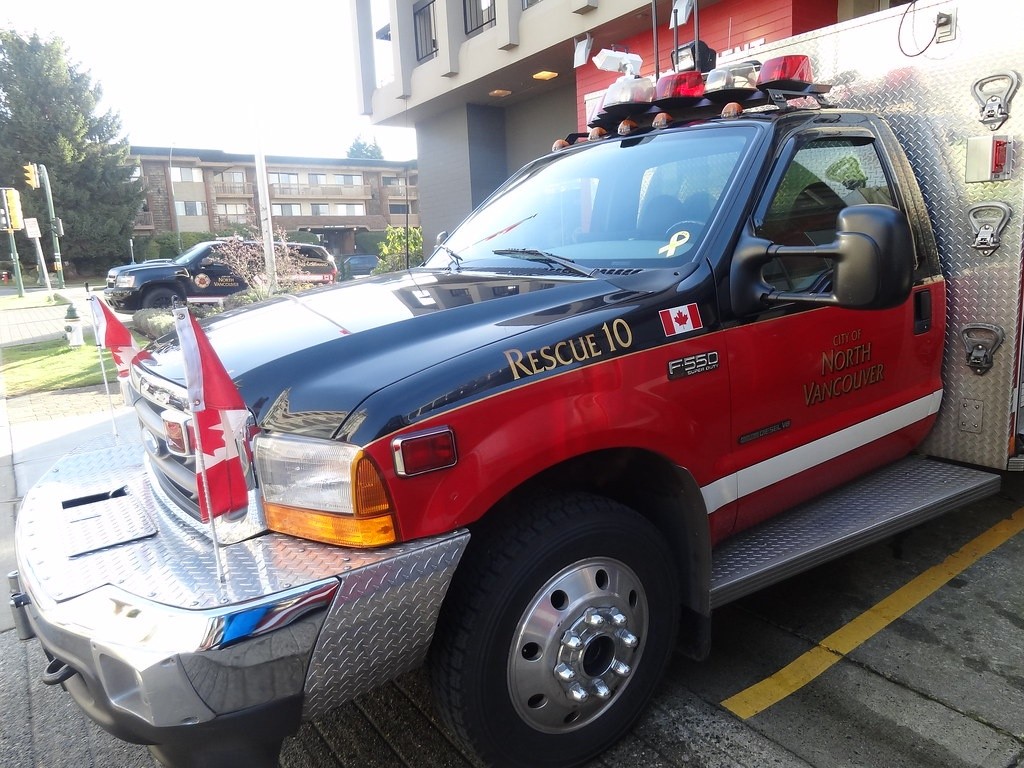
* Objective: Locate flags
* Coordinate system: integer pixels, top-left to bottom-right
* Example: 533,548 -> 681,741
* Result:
171,307 -> 260,525
90,294 -> 158,378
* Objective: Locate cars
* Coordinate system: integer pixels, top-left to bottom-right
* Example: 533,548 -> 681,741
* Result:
0,260 -> 25,281
338,254 -> 380,276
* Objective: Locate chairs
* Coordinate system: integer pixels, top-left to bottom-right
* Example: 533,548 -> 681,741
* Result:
778,182 -> 855,278
637,195 -> 689,241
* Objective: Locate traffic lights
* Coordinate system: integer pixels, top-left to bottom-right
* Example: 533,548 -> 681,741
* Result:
24,163 -> 40,191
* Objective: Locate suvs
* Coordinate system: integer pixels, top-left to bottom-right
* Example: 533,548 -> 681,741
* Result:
104,240 -> 339,316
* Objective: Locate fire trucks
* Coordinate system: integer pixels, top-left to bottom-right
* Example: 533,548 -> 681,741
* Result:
6,0 -> 1024,768
